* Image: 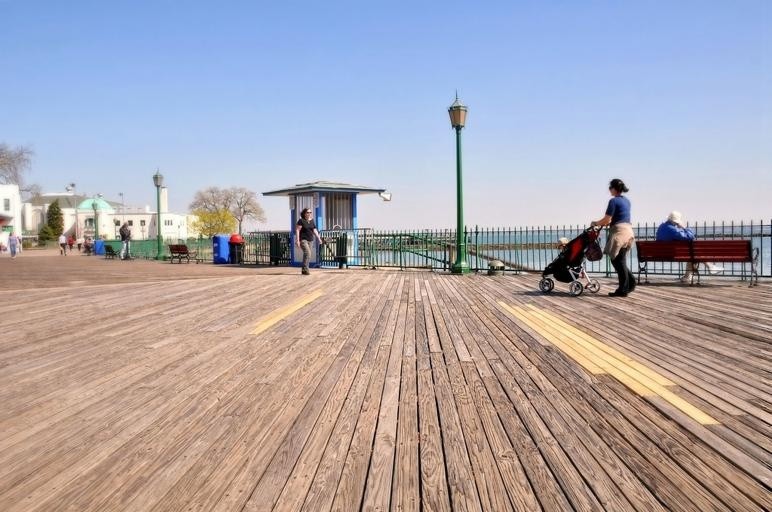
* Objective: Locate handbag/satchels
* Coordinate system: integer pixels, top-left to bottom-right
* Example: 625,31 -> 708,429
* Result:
585,241 -> 602,261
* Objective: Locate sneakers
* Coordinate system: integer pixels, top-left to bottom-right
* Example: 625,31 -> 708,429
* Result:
608,291 -> 627,297
679,275 -> 693,284
708,267 -> 724,275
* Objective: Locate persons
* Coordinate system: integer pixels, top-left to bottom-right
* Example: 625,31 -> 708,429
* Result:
119,221 -> 131,260
58,232 -> 92,256
591,178 -> 636,297
656,213 -> 724,282
7,232 -> 18,258
295,208 -> 323,274
556,237 -> 568,251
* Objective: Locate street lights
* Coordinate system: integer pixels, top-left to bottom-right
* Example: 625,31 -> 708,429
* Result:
113,218 -> 117,240
65,183 -> 80,238
117,192 -> 125,222
91,192 -> 104,227
152,167 -> 166,260
90,199 -> 99,240
447,87 -> 471,275
141,223 -> 144,240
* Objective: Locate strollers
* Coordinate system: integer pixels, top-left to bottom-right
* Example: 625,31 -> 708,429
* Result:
535,224 -> 605,297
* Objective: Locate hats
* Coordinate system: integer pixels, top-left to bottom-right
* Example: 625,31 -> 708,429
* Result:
667,211 -> 682,224
557,237 -> 569,249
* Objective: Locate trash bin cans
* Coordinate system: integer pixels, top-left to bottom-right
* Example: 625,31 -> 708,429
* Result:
93,240 -> 105,256
211,234 -> 230,264
227,232 -> 245,264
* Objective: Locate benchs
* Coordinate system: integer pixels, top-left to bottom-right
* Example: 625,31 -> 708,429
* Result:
104,245 -> 117,259
635,241 -> 699,286
691,239 -> 759,287
168,245 -> 199,264
